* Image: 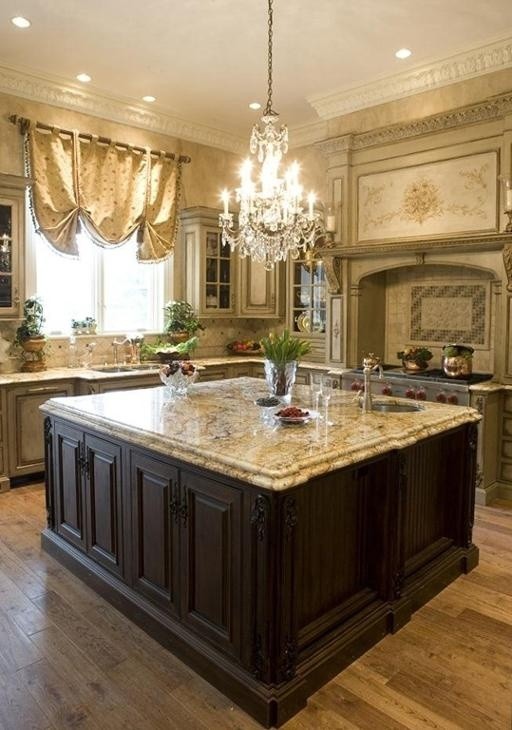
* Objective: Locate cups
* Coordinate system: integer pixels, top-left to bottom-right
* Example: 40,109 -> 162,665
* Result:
264,359 -> 297,404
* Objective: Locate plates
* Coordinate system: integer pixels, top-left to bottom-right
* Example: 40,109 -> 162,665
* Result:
296,311 -> 320,332
272,405 -> 318,425
228,345 -> 269,356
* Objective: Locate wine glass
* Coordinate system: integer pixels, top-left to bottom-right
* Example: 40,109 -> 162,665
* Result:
308,372 -> 337,425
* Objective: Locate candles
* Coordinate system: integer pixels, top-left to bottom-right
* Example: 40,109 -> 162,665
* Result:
506,180 -> 512,211
326,208 -> 337,234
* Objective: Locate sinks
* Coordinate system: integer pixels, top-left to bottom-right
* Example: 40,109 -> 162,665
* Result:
137,362 -> 169,371
371,398 -> 424,413
93,362 -> 134,372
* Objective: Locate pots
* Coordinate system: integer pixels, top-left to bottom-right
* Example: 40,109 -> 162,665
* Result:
441,355 -> 473,379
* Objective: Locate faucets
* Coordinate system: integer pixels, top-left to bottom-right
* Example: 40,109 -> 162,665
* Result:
111,336 -> 136,362
363,358 -> 386,413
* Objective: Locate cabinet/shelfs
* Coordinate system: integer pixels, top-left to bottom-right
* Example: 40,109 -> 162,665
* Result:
0,194 -> 25,318
176,206 -> 286,319
290,249 -> 328,339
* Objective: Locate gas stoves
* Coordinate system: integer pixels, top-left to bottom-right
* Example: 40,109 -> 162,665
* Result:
385,365 -> 493,386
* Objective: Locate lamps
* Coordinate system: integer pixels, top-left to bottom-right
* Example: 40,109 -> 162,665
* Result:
218,0 -> 326,272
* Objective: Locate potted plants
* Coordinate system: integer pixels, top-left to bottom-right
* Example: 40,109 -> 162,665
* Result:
163,300 -> 207,359
6,295 -> 48,373
70,317 -> 98,335
259,328 -> 313,395
396,346 -> 433,375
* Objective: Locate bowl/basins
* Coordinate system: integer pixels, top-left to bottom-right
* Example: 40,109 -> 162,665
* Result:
401,359 -> 429,373
159,369 -> 196,395
